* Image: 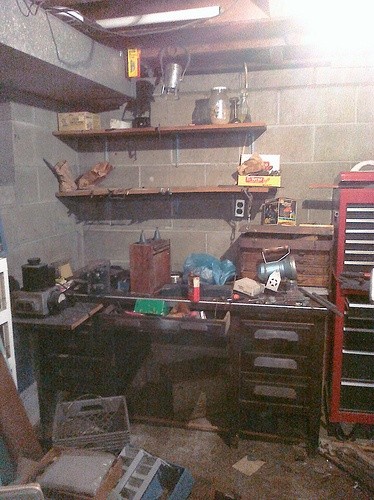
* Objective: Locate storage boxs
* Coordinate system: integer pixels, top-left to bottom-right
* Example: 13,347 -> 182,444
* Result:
237,153 -> 281,188
0,352 -> 228,500
130,238 -> 171,295
58,111 -> 101,131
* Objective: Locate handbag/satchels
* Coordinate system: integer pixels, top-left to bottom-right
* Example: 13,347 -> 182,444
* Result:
182,253 -> 236,287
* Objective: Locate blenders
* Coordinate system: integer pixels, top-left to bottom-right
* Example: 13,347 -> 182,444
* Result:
132,80 -> 154,128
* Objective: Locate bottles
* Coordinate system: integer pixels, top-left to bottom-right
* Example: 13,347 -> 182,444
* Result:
237,92 -> 249,123
208,86 -> 230,124
192,99 -> 211,125
187,272 -> 200,302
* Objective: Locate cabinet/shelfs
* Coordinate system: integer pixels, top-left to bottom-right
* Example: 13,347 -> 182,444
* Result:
307,171 -> 374,440
9,280 -> 330,458
237,225 -> 335,288
52,122 -> 269,197
